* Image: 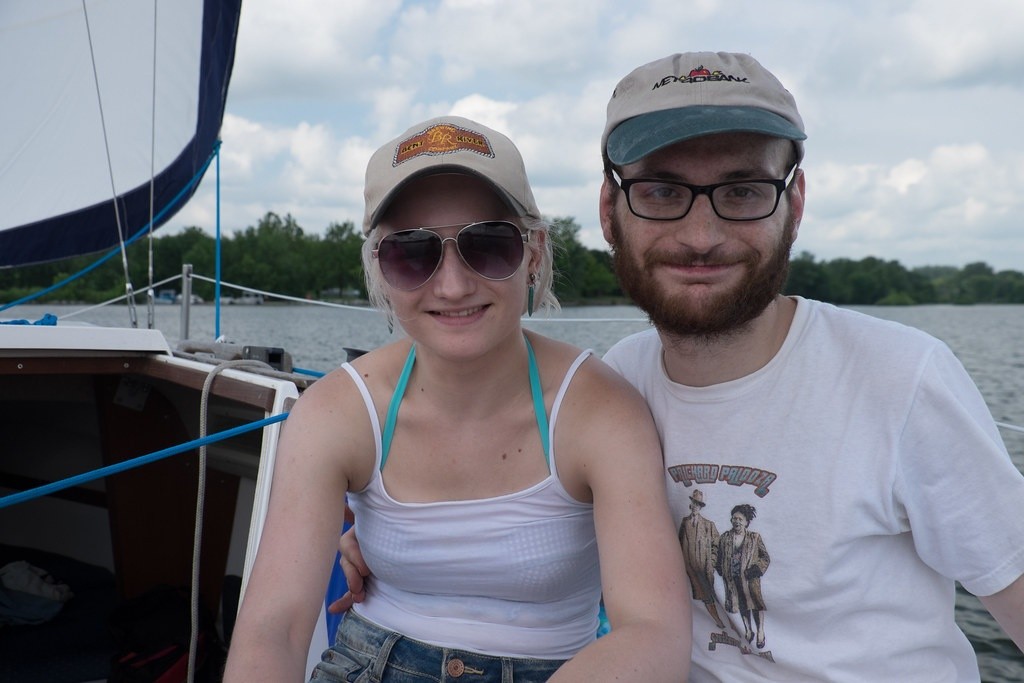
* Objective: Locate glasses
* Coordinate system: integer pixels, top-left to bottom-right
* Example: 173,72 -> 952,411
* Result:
611,164 -> 796,221
371,219 -> 530,292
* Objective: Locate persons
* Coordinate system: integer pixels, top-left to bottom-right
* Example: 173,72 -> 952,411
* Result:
328,50 -> 1024,683
223,116 -> 693,682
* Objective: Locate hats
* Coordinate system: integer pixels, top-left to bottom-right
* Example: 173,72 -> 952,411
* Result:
361,116 -> 540,235
600,51 -> 806,176
